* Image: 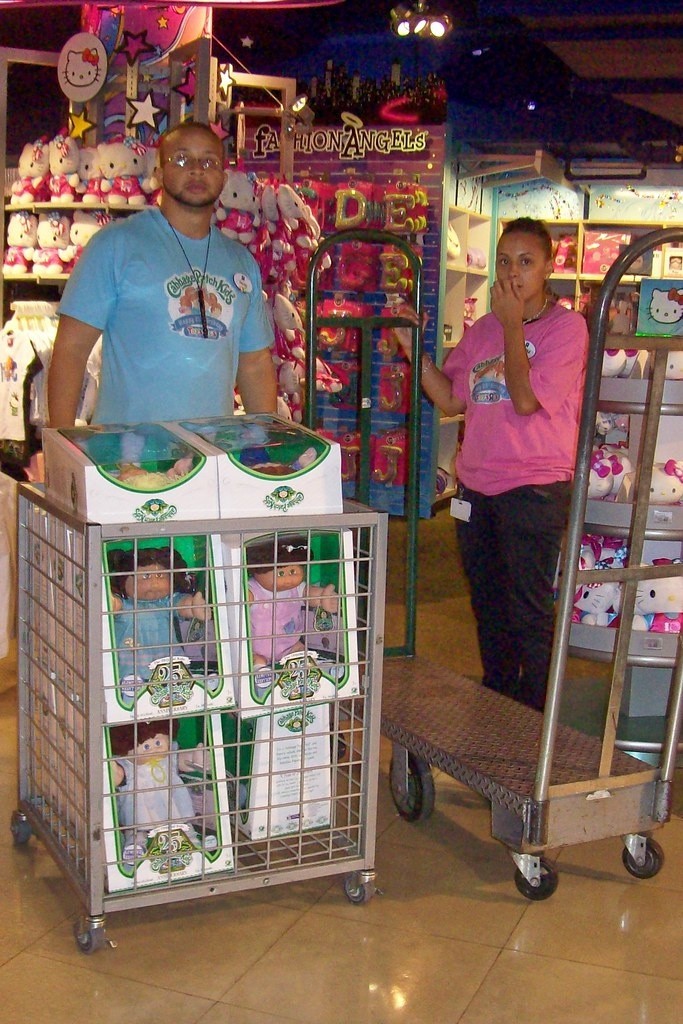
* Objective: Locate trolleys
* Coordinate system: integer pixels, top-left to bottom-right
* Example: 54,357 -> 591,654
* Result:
13,481 -> 390,957
303,226 -> 683,902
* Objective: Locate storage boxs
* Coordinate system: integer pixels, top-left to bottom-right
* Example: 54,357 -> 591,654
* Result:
583,230 -> 639,275
41,421 -> 236,723
41,672 -> 234,895
173,411 -> 361,720
220,703 -> 333,841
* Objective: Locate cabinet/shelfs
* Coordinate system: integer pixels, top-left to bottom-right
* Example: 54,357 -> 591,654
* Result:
433,203 -> 683,502
565,375 -> 683,768
3,202 -> 147,294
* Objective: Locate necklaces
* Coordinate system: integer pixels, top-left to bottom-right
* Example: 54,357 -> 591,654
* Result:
522,298 -> 548,325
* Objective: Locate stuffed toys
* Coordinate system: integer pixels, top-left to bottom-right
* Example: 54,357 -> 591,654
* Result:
0,126 -> 683,898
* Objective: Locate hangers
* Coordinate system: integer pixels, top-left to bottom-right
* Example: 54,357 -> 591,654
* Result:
1,301 -> 60,337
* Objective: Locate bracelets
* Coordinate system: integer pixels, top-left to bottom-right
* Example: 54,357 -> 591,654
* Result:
422,355 -> 432,374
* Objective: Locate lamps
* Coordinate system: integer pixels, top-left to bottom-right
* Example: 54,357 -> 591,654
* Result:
292,94 -> 315,126
390,0 -> 454,39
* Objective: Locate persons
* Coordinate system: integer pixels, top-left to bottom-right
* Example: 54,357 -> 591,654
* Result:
48,124 -> 277,429
393,217 -> 590,714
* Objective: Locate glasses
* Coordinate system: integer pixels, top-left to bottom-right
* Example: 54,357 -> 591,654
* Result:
158,155 -> 226,172
